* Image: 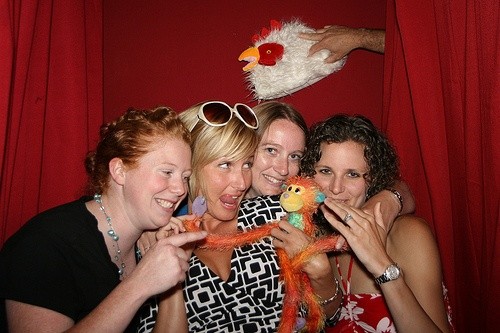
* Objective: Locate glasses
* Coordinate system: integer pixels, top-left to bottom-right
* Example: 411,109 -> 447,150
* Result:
189,100 -> 260,133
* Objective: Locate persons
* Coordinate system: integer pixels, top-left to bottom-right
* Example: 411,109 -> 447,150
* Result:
0,99 -> 456,333
297,24 -> 386,64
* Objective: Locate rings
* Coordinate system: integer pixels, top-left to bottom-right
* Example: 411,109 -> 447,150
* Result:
343,214 -> 352,222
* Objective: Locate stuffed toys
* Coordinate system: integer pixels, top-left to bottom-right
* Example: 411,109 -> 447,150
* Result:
237,16 -> 347,101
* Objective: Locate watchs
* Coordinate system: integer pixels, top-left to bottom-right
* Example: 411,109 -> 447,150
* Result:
374,263 -> 401,285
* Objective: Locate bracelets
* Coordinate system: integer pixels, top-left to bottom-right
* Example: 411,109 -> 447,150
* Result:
324,301 -> 342,327
384,188 -> 404,216
318,276 -> 339,304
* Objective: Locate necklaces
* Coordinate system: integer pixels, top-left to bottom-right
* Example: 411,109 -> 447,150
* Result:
93,192 -> 130,282
